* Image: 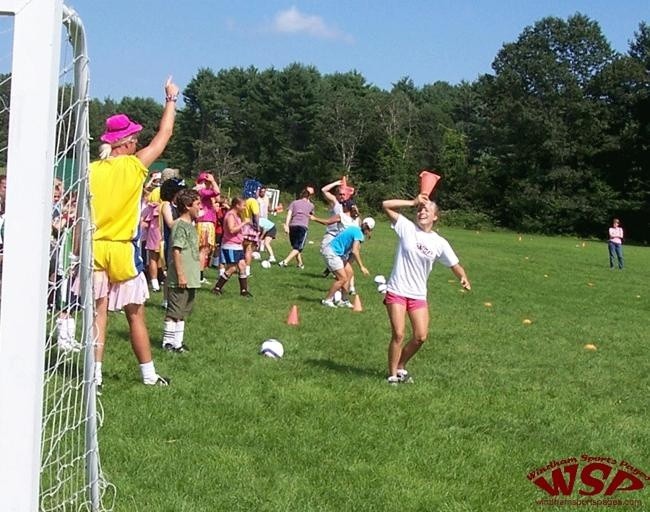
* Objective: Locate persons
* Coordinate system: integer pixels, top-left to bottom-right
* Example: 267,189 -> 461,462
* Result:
1,146 -> 376,355
379,192 -> 471,388
70,73 -> 180,397
606,218 -> 625,271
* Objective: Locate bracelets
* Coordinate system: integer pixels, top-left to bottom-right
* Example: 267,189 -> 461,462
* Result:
166,94 -> 178,102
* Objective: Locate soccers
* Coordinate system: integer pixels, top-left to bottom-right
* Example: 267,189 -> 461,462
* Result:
374,275 -> 388,294
260,338 -> 284,360
261,260 -> 271,269
252,252 -> 261,260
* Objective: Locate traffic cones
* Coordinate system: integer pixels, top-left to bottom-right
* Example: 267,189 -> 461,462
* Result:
415,171 -> 440,200
284,305 -> 304,327
336,175 -> 348,190
351,293 -> 363,314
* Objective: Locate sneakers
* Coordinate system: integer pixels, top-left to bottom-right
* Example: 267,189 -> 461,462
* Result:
240,289 -> 254,298
160,341 -> 191,354
58,335 -> 84,353
143,374 -> 171,387
337,299 -> 354,308
388,375 -> 398,382
278,260 -> 288,269
398,368 -> 409,383
320,298 -> 338,309
267,256 -> 277,262
349,291 -> 356,296
211,286 -> 223,296
296,264 -> 305,270
95,382 -> 103,396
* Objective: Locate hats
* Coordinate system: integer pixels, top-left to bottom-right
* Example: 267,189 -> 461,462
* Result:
196,171 -> 209,184
361,216 -> 376,229
98,113 -> 144,143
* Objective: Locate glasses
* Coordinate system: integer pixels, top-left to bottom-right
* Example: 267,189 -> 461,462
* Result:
338,193 -> 346,196
